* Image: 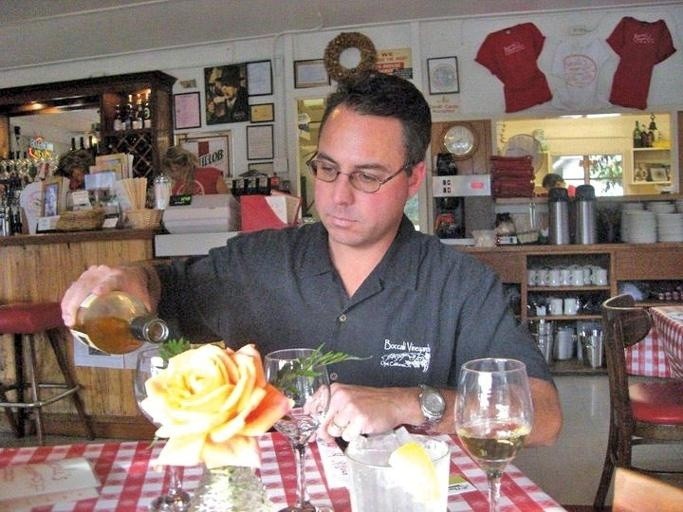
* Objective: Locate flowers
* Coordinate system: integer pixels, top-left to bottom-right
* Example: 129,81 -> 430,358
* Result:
139,344 -> 295,470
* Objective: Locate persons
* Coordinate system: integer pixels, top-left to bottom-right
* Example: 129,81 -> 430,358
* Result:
162,146 -> 232,195
207,65 -> 249,125
541,172 -> 577,198
61,70 -> 564,449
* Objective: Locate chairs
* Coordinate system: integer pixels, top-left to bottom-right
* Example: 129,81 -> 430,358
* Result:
593,295 -> 682,511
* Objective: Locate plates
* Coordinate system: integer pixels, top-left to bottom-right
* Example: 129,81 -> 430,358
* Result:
621,210 -> 655,246
658,213 -> 682,241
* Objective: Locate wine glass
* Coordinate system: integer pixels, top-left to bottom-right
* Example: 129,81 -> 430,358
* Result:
135,350 -> 190,512
264,348 -> 331,511
454,357 -> 534,511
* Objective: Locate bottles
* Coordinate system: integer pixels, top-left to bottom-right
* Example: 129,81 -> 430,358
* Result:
134,94 -> 144,130
549,188 -> 571,245
123,94 -> 133,129
575,186 -> 597,245
70,290 -> 169,354
143,93 -> 151,129
0,149 -> 33,182
634,121 -> 642,148
112,104 -> 121,131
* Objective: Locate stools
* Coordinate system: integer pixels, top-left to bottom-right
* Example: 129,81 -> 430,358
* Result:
0,302 -> 96,446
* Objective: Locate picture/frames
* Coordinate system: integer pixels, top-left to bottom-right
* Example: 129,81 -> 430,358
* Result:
174,91 -> 201,130
294,59 -> 331,88
246,59 -> 272,97
247,125 -> 273,159
249,102 -> 274,122
178,135 -> 230,178
427,58 -> 460,96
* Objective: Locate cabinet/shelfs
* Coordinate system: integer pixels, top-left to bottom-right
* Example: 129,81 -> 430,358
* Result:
446,244 -> 682,378
630,109 -> 671,188
1,71 -> 176,205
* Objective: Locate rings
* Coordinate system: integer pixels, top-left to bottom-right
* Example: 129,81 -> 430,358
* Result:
330,420 -> 345,434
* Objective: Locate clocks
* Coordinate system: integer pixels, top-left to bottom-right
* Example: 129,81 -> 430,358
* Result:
437,122 -> 480,163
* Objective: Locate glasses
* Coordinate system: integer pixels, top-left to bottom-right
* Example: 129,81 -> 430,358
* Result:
305,152 -> 411,192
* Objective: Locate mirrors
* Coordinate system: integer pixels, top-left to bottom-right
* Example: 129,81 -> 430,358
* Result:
8,107 -> 100,160
494,107 -> 679,199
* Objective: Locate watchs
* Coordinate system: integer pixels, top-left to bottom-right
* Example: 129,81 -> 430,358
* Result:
408,382 -> 447,434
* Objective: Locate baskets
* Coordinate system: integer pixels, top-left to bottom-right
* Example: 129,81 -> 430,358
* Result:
128,209 -> 162,231
57,211 -> 103,231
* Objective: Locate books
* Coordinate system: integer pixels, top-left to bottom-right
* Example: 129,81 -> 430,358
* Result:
85,153 -> 133,188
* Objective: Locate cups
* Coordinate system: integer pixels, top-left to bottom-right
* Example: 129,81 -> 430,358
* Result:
154,175 -> 170,211
549,299 -> 564,315
564,299 -> 580,315
526,265 -> 610,289
343,436 -> 450,512
